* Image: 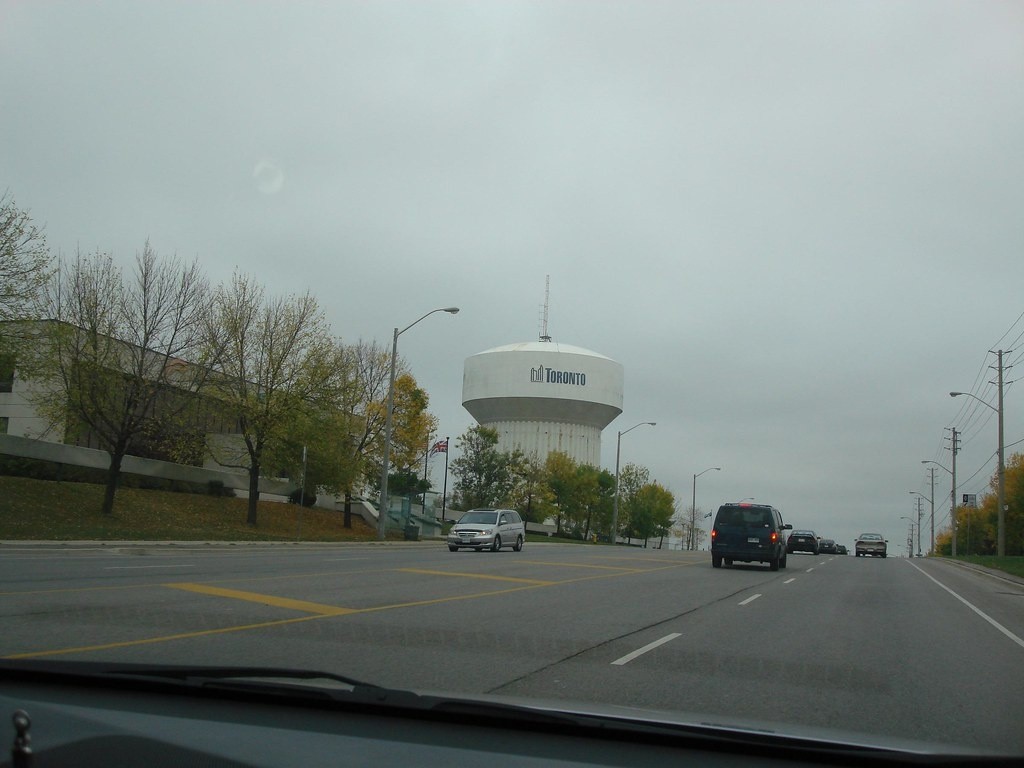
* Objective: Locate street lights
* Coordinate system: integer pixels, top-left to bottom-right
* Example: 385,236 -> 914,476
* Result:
909,491 -> 935,554
376,305 -> 461,541
920,460 -> 956,557
901,516 -> 921,557
691,467 -> 721,551
949,390 -> 1006,556
611,422 -> 658,545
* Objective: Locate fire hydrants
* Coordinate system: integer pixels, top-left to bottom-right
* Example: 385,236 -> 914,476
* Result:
591,533 -> 598,544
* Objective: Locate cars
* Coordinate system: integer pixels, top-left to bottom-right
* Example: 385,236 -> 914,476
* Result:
786,529 -> 821,555
446,508 -> 525,552
854,532 -> 889,559
818,538 -> 850,555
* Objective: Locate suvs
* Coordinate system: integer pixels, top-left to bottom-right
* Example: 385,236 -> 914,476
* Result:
710,502 -> 793,572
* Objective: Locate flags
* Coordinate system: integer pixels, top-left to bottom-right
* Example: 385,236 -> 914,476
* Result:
430,441 -> 447,457
